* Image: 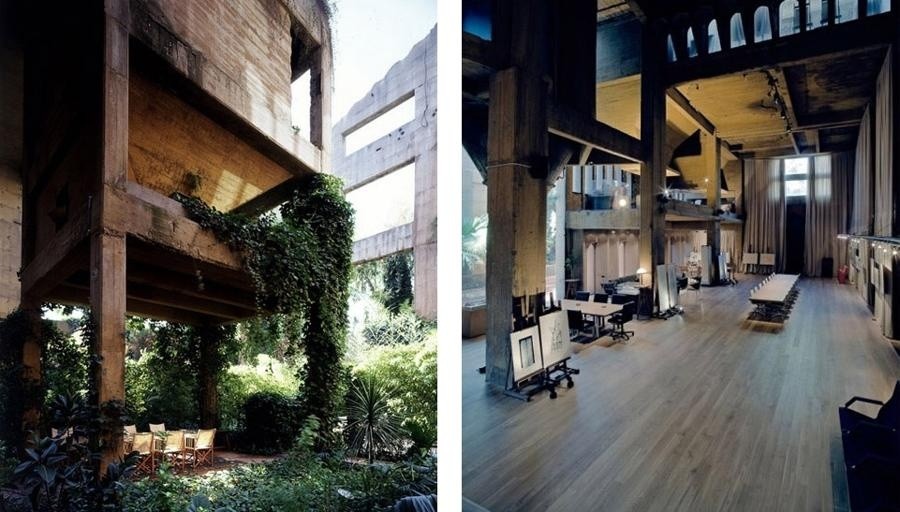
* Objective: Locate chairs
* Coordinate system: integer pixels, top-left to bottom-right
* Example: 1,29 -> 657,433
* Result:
124,423 -> 217,475
689,275 -> 702,298
748,270 -> 801,321
546,291 -> 636,340
837,378 -> 900,512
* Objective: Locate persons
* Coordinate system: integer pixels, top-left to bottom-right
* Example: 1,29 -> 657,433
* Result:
550,318 -> 563,353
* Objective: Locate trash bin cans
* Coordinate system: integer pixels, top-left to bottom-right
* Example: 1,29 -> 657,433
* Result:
837,271 -> 846,284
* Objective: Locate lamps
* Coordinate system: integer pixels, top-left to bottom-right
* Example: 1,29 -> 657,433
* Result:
773,92 -> 791,133
636,268 -> 647,286
